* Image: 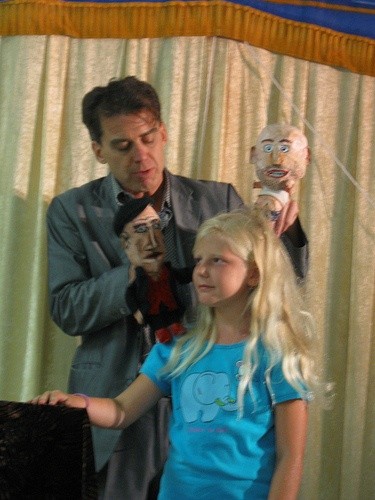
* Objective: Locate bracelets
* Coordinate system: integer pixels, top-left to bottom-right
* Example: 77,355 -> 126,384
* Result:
72,392 -> 90,411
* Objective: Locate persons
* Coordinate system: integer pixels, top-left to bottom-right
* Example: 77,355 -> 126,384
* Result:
46,77 -> 308,500
111,193 -> 195,344
25,208 -> 337,500
247,124 -> 312,237
